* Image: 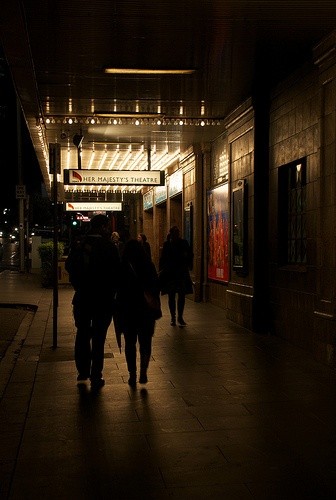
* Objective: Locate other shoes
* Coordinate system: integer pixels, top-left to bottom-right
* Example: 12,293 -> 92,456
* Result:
177,317 -> 186,326
91,379 -> 105,391
76,370 -> 91,381
170,318 -> 176,326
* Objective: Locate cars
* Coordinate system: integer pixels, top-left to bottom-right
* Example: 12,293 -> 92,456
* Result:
0,227 -> 54,258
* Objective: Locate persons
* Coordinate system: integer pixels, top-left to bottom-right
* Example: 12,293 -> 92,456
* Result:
110,232 -> 124,256
137,233 -> 151,254
158,226 -> 194,327
64,214 -> 162,389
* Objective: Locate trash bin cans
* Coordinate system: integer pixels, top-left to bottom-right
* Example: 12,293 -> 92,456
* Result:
57,256 -> 71,285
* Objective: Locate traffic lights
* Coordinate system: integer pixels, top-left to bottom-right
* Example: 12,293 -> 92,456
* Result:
72,220 -> 81,231
